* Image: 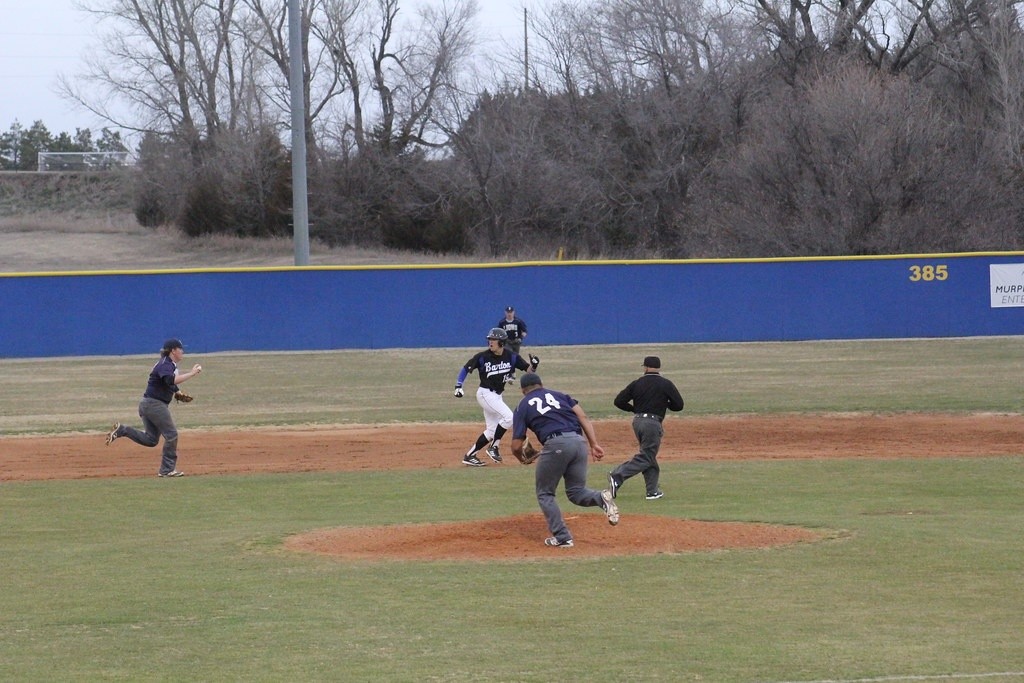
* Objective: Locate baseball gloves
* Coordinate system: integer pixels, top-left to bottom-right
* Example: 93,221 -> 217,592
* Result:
520,437 -> 541,465
174,393 -> 193,402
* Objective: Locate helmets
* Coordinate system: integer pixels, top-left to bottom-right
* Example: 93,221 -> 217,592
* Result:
487,327 -> 508,340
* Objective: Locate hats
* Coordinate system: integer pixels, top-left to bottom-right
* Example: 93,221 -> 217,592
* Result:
520,373 -> 542,387
163,338 -> 188,348
641,356 -> 661,368
506,306 -> 514,311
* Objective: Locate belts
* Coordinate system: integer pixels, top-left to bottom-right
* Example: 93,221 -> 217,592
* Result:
546,431 -> 580,438
634,413 -> 662,423
481,386 -> 501,394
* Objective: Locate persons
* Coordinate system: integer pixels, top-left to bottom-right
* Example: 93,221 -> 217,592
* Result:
455,327 -> 540,467
498,307 -> 527,384
105,338 -> 202,477
511,373 -> 619,548
607,356 -> 684,499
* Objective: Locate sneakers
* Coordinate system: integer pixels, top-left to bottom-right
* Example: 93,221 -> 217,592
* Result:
607,470 -> 621,498
158,469 -> 184,477
486,444 -> 503,463
600,490 -> 619,525
508,377 -> 515,385
105,422 -> 125,446
544,537 -> 574,547
462,454 -> 486,466
646,491 -> 664,499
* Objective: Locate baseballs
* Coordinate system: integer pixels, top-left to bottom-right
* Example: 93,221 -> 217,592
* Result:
197,365 -> 202,370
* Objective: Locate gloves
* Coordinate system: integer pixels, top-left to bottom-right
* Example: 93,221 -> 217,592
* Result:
518,337 -> 524,342
529,354 -> 539,371
454,386 -> 464,397
